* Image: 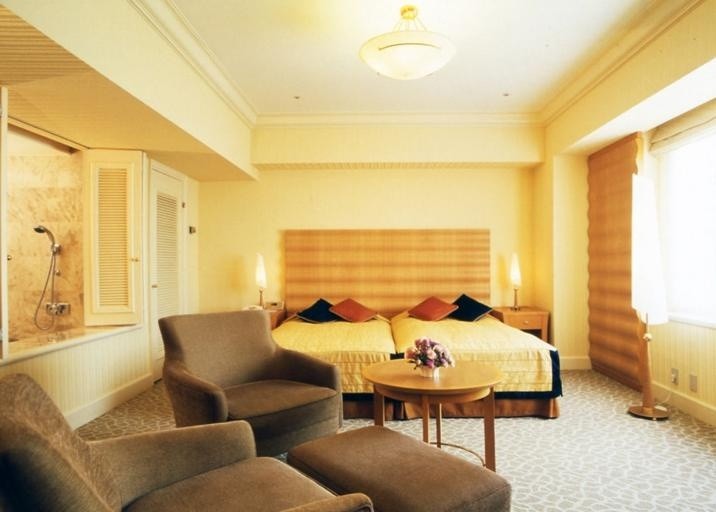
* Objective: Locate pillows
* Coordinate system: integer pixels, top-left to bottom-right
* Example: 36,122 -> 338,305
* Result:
408,296 -> 459,322
446,293 -> 493,322
328,298 -> 379,323
295,298 -> 341,324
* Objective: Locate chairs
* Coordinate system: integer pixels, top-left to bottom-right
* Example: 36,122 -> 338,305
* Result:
157,309 -> 345,458
0,373 -> 375,511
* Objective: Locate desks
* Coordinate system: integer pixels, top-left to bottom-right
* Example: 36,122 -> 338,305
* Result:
363,359 -> 505,474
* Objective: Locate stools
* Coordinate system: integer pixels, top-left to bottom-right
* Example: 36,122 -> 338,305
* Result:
286,425 -> 512,511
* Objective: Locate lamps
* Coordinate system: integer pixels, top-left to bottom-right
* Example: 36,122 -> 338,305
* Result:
358,6 -> 457,82
627,171 -> 672,422
254,253 -> 268,309
509,251 -> 523,310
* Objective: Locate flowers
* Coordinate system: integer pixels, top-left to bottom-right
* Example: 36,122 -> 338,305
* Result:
405,337 -> 455,369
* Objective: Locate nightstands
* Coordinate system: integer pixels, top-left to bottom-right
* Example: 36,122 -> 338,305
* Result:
491,305 -> 549,343
244,303 -> 287,331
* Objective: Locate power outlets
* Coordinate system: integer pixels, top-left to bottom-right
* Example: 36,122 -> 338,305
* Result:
672,368 -> 679,385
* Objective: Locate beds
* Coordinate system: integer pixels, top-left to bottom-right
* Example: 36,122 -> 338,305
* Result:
389,306 -> 564,420
271,314 -> 398,421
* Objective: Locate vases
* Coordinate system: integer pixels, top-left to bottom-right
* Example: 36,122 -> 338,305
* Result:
418,365 -> 440,379
